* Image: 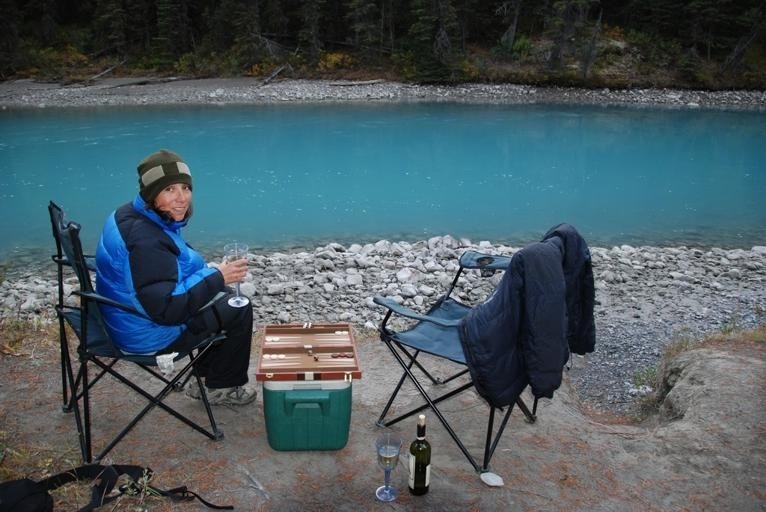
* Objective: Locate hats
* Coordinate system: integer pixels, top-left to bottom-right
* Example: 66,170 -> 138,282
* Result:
137,149 -> 191,201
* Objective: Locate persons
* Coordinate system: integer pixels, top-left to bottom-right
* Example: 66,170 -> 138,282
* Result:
94,150 -> 259,405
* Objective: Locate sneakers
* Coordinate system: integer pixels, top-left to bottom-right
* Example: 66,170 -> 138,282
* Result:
186,380 -> 205,400
205,387 -> 257,405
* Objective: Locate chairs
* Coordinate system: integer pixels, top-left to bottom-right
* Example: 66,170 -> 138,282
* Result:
47,199 -> 229,467
373,219 -> 580,471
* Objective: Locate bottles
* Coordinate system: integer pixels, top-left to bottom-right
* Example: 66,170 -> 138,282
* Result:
405,413 -> 438,498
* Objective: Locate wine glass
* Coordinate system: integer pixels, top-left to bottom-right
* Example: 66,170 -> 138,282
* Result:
374,433 -> 403,504
222,241 -> 252,311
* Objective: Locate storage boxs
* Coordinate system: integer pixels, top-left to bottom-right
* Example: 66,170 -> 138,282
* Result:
261,378 -> 353,451
